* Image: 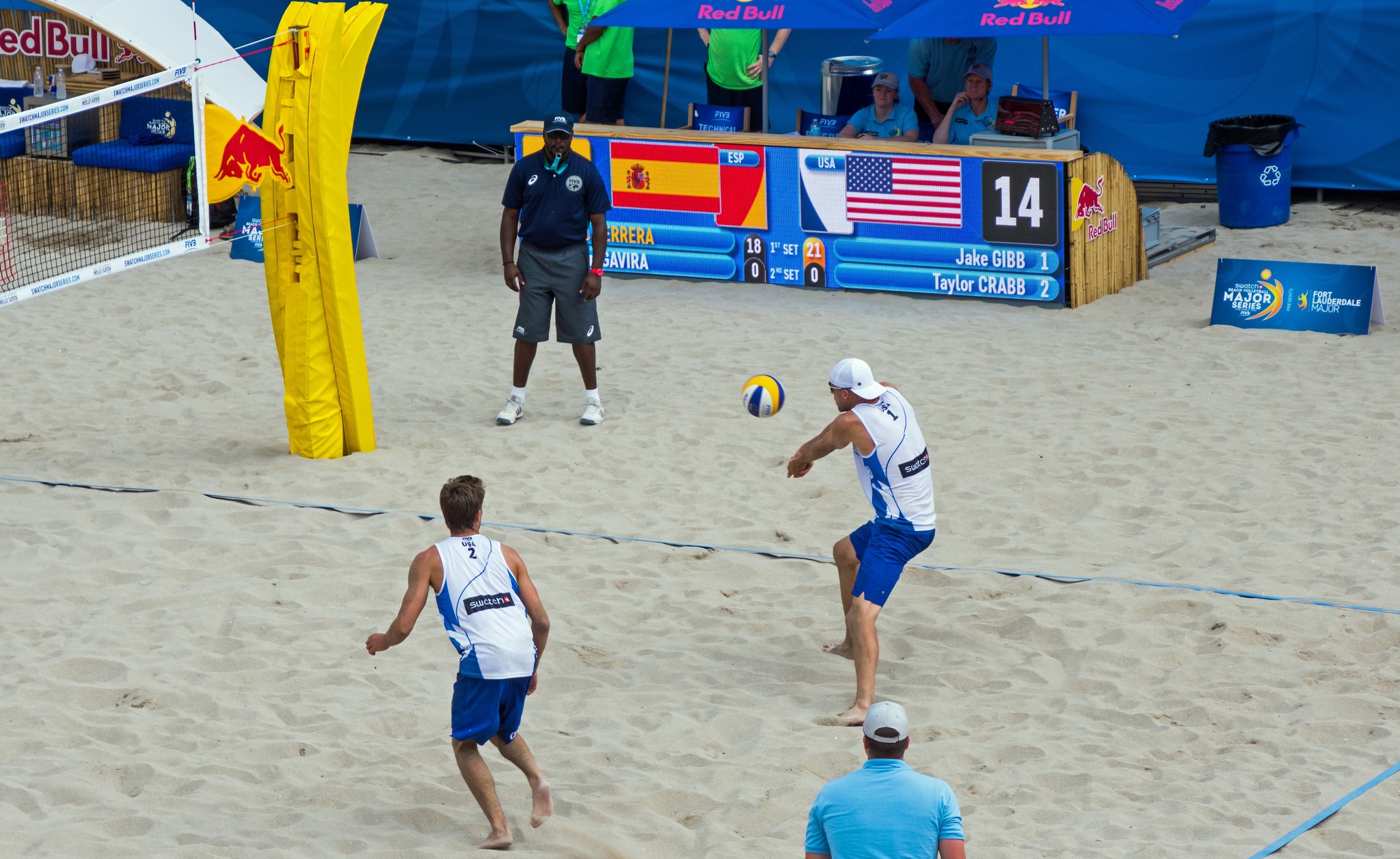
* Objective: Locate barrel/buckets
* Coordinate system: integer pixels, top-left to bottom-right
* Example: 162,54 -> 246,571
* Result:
820,55 -> 883,116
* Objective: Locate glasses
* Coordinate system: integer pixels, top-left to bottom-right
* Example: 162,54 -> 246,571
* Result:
547,131 -> 570,140
828,381 -> 853,392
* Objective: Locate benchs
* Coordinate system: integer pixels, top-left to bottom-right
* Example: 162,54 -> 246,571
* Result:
0,86 -> 48,160
72,95 -> 197,175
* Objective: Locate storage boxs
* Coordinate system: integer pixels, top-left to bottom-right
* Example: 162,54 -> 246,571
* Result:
1142,200 -> 1162,251
968,128 -> 1080,151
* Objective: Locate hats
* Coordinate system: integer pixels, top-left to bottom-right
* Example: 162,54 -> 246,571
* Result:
863,701 -> 908,743
830,357 -> 887,400
543,110 -> 574,134
871,72 -> 899,89
963,62 -> 993,82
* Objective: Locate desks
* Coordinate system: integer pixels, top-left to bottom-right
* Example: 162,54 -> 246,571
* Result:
509,119 -> 1150,312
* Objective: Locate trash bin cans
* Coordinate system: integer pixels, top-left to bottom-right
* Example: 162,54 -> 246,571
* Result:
1208,114 -> 1300,229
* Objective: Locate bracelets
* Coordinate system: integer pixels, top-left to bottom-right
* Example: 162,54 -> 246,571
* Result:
503,261 -> 514,266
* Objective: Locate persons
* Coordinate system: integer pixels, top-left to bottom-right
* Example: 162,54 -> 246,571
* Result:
590,268 -> 604,276
549,0 -> 607,123
574,0 -> 635,126
696,28 -> 791,132
365,475 -> 553,850
932,62 -> 1000,145
804,700 -> 966,859
907,36 -> 998,143
838,71 -> 919,143
496,110 -> 611,425
788,358 -> 936,727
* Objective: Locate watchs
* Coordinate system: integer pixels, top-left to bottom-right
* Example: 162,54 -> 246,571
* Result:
768,51 -> 777,59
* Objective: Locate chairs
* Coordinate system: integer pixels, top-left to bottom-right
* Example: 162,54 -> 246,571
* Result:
1012,83 -> 1092,157
796,108 -> 853,138
675,102 -> 751,133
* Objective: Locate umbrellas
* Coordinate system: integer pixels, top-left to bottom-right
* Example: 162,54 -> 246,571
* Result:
864,0 -> 1211,99
587,0 -> 929,134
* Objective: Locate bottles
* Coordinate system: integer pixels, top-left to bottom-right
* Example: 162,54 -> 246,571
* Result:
56,69 -> 67,99
34,67 -> 44,97
810,119 -> 821,137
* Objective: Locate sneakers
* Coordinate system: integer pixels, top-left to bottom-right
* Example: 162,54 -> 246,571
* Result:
580,397 -> 604,425
496,395 -> 524,425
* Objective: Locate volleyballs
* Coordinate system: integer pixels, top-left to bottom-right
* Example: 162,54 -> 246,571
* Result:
741,374 -> 786,419
